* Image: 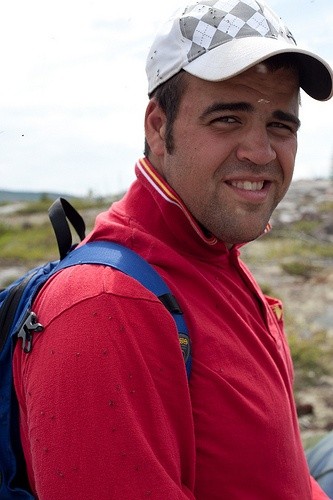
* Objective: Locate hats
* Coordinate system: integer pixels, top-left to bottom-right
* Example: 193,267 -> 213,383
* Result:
146,0 -> 333,101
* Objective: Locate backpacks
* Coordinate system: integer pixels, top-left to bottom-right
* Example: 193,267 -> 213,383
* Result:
0,197 -> 193,500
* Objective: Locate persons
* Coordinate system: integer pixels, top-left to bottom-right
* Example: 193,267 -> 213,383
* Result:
0,0 -> 333,500
